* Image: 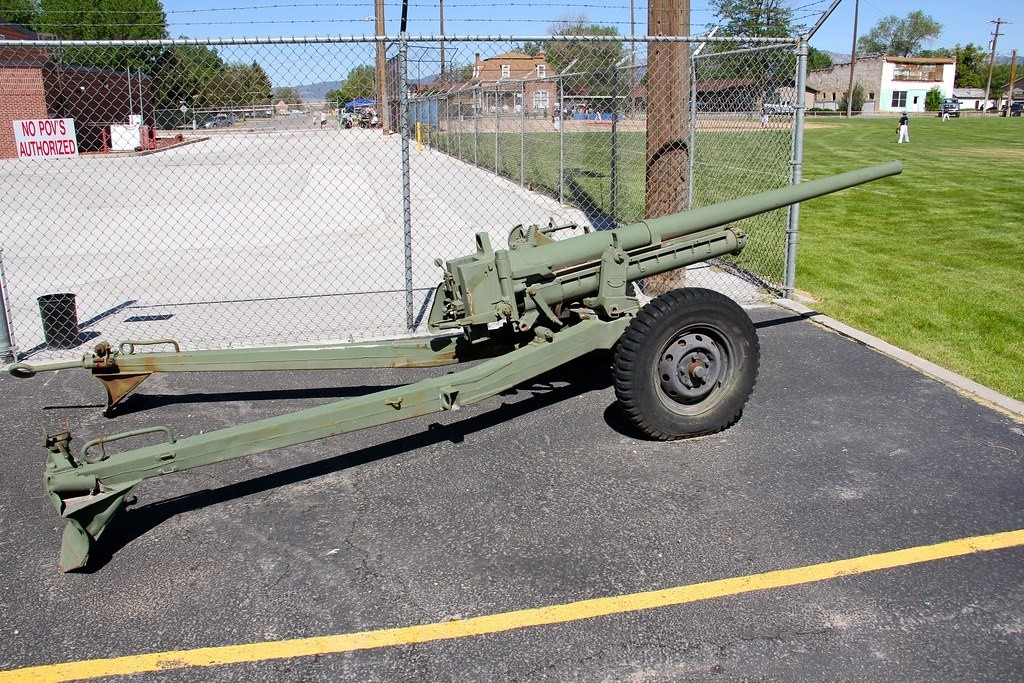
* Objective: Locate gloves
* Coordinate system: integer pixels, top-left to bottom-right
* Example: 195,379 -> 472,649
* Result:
896,128 -> 899,133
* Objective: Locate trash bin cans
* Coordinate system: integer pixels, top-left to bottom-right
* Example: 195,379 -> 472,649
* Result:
37,293 -> 79,351
138,126 -> 157,151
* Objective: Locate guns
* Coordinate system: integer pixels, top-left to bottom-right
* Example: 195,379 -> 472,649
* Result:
419,160 -> 907,334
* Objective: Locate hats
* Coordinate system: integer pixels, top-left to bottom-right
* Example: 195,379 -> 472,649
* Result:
903,110 -> 907,114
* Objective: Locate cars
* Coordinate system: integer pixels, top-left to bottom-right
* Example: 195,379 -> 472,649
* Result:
1002,104 -> 1024,117
762,100 -> 810,115
202,116 -> 233,129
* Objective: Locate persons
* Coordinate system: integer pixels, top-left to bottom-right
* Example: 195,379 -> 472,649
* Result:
897,111 -> 911,144
310,105 -> 379,132
979,101 -> 1006,112
942,106 -> 951,123
472,101 -> 604,130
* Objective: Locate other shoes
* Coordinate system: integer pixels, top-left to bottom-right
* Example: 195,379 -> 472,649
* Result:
897,142 -> 902,143
904,141 -> 909,143
594,121 -> 597,123
600,121 -> 603,122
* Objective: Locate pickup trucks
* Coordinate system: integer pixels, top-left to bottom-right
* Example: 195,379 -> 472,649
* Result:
938,98 -> 963,117
